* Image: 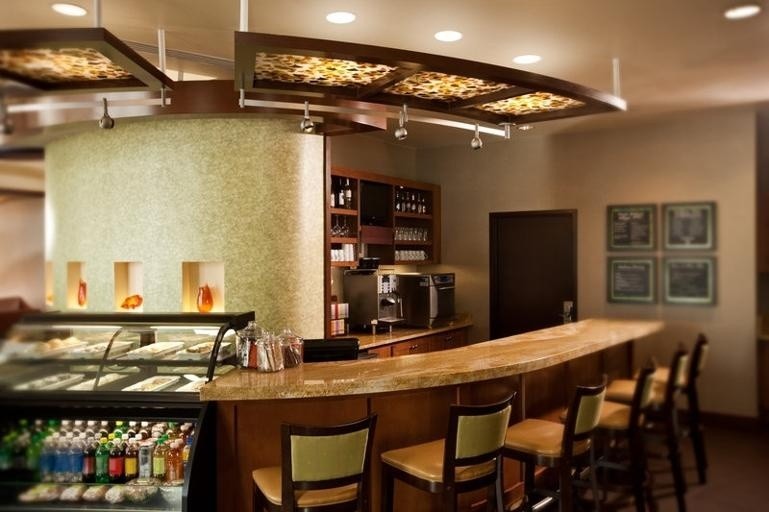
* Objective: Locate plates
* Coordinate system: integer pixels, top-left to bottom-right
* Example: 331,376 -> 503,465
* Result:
17,338 -> 91,360
12,373 -> 84,391
125,341 -> 184,360
67,373 -> 127,392
177,341 -> 233,359
74,341 -> 133,360
175,375 -> 217,395
121,375 -> 180,392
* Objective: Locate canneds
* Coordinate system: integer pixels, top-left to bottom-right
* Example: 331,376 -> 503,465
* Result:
138,424 -> 167,477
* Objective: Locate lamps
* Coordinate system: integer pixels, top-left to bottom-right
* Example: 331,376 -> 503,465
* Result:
298,103 -> 486,150
0,100 -> 121,138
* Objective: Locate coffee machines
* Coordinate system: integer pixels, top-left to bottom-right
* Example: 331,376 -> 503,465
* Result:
344,267 -> 406,335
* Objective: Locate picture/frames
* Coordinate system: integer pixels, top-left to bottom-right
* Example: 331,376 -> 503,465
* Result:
661,200 -> 716,251
662,255 -> 717,305
606,255 -> 658,305
605,202 -> 658,253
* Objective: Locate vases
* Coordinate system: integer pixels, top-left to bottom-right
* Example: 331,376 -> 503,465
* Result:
77,280 -> 87,307
196,282 -> 214,314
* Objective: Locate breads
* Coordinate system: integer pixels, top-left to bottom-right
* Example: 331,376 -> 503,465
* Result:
19,484 -> 124,503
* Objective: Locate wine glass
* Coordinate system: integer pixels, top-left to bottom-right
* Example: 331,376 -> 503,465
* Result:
331,213 -> 350,238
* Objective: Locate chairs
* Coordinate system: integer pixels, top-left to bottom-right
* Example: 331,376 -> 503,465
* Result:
557,354 -> 660,511
632,331 -> 711,485
501,373 -> 610,511
378,390 -> 520,512
249,410 -> 379,511
601,340 -> 691,511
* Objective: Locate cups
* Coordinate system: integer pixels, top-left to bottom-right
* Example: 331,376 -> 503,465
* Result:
331,243 -> 355,262
395,250 -> 428,260
395,226 -> 428,242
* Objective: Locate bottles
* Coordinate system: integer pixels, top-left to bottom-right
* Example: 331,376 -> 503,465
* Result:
1,420 -> 196,484
235,320 -> 271,370
330,177 -> 352,210
196,283 -> 213,312
76,278 -> 88,307
276,321 -> 303,368
395,189 -> 427,214
254,330 -> 284,373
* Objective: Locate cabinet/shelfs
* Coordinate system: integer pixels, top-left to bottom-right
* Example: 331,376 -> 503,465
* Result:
0,311 -> 258,512
368,328 -> 468,357
330,165 -> 441,267
